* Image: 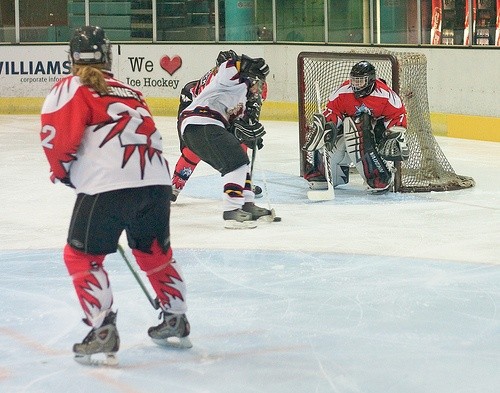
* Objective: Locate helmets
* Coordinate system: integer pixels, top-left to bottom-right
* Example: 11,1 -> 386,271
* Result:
257,26 -> 273,40
286,30 -> 304,42
349,60 -> 376,98
70,27 -> 112,64
216,49 -> 238,69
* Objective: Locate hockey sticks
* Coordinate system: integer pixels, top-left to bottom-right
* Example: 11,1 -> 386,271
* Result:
117,240 -> 161,310
250,148 -> 256,178
306,80 -> 336,202
256,144 -> 276,219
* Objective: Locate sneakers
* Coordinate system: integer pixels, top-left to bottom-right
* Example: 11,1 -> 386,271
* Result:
73,310 -> 121,365
171,183 -> 181,206
148,310 -> 194,349
222,208 -> 259,230
243,200 -> 276,224
246,172 -> 263,198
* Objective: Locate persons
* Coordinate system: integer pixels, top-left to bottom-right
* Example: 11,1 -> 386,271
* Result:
302,60 -> 409,195
40,25 -> 190,355
171,49 -> 271,223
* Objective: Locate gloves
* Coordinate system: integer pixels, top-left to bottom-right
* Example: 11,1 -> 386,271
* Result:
240,54 -> 270,81
232,118 -> 266,142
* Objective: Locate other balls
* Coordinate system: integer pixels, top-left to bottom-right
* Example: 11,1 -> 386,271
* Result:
273,216 -> 281,222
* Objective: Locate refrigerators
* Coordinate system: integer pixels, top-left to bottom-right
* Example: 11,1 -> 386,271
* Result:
420,0 -> 500,45
